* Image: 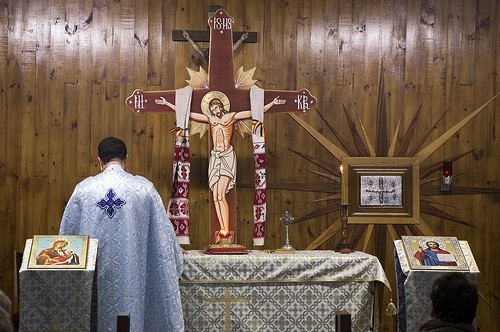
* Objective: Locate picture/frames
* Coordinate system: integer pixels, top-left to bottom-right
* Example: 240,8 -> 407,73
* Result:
27,234 -> 90,269
402,235 -> 471,271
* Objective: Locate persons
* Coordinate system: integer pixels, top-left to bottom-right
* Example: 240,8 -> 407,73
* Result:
59,137 -> 184,332
418,273 -> 482,332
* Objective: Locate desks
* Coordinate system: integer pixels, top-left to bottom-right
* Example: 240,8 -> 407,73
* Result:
180,250 -> 398,332
